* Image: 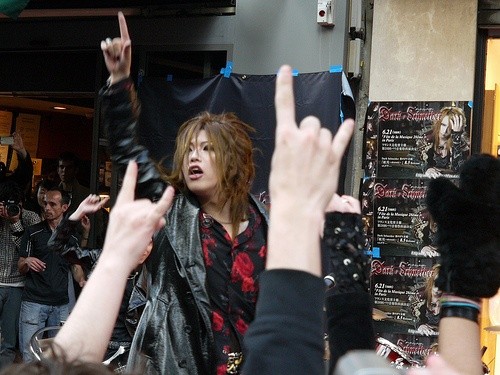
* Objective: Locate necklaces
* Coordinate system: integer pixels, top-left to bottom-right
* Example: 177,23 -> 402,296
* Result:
220,221 -> 236,226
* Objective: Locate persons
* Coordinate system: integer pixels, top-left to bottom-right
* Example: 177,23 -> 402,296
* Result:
101,13 -> 269,375
0,132 -> 174,375
238,63 -> 500,375
417,204 -> 441,258
421,106 -> 469,179
415,265 -> 441,337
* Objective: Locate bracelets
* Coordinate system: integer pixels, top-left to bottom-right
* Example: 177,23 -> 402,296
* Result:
438,307 -> 480,325
438,294 -> 481,310
82,237 -> 88,241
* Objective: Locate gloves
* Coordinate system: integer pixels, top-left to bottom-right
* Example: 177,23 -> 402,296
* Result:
424,153 -> 500,303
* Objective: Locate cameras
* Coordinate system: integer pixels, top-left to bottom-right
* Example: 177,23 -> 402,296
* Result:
2,199 -> 20,217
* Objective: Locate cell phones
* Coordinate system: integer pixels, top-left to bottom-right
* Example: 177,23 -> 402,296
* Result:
0,136 -> 14,145
